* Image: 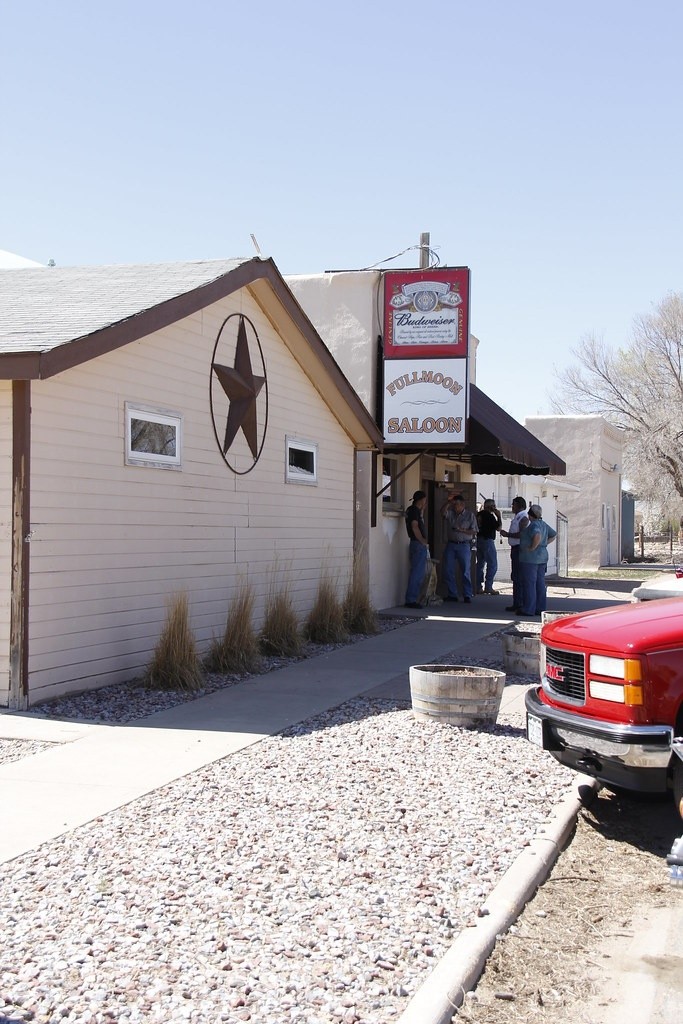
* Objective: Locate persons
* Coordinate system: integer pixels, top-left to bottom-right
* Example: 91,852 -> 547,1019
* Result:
476,499 -> 502,595
500,496 -> 557,616
441,494 -> 479,605
403,491 -> 430,610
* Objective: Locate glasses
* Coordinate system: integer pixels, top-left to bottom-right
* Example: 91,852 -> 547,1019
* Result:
488,505 -> 496,507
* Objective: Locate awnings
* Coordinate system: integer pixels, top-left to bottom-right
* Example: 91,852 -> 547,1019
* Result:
371,335 -> 566,529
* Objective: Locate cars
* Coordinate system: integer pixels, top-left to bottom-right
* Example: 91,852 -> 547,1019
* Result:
631,569 -> 682,604
522,596 -> 682,806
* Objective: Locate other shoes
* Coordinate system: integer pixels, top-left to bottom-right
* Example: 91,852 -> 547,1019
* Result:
475,588 -> 499,595
442,595 -> 458,602
404,602 -> 422,609
515,610 -> 534,617
534,609 -> 546,616
464,595 -> 471,603
505,605 -> 517,612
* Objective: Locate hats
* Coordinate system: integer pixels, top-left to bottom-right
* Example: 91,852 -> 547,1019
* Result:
409,491 -> 426,502
528,505 -> 542,519
448,496 -> 464,502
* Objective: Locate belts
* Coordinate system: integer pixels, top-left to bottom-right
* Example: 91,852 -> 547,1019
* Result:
449,540 -> 466,544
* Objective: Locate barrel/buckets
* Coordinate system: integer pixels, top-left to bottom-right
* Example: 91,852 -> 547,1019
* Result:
541,610 -> 579,628
502,632 -> 540,675
409,664 -> 506,728
